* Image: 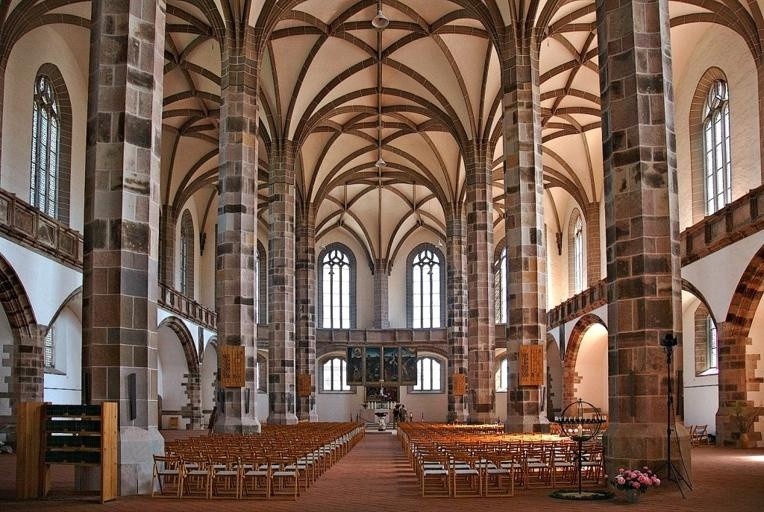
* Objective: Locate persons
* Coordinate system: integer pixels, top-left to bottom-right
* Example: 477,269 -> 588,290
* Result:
398,404 -> 407,422
392,404 -> 399,429
349,347 -> 416,382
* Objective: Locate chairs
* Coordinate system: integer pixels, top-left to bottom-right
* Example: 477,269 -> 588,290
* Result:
395,420 -> 606,499
692,424 -> 709,448
151,417 -> 365,501
684,425 -> 695,448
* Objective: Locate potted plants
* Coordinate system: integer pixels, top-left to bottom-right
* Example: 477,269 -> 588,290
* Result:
726,403 -> 762,449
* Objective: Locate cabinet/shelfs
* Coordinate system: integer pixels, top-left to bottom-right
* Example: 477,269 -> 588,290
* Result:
39,401 -> 118,504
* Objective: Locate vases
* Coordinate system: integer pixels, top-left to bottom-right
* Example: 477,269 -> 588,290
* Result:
625,489 -> 641,502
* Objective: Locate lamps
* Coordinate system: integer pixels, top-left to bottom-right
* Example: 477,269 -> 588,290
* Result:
375,156 -> 385,167
372,10 -> 390,28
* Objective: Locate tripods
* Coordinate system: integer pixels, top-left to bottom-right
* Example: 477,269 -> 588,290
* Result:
649,344 -> 692,498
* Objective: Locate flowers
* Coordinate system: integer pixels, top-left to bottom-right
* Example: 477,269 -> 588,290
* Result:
613,465 -> 661,497
